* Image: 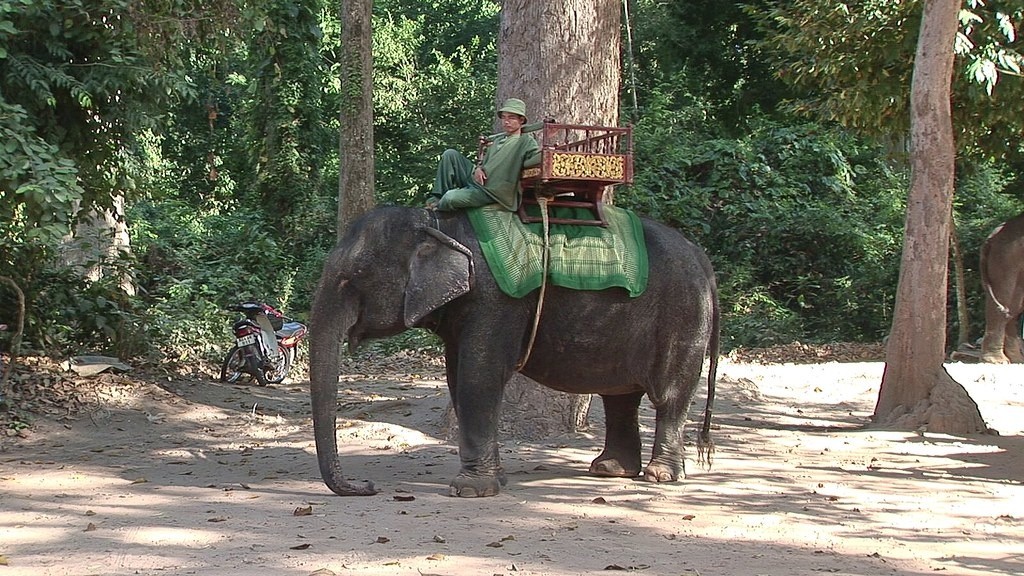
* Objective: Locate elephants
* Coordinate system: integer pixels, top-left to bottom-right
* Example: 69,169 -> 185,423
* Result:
979,212 -> 1024,364
308,195 -> 721,497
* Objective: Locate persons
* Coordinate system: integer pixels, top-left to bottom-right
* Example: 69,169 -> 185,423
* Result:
426,98 -> 539,211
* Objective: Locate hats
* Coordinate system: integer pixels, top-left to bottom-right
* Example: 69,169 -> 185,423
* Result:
496,97 -> 528,126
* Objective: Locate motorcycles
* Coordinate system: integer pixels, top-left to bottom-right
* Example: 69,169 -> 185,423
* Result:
220,300 -> 308,384
